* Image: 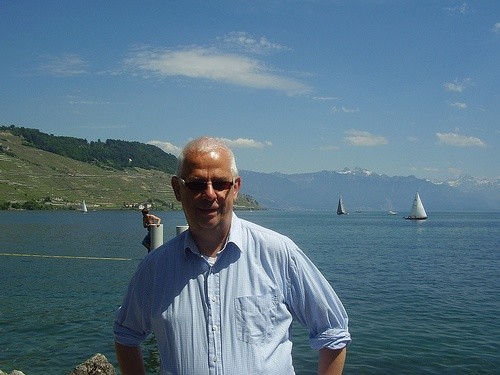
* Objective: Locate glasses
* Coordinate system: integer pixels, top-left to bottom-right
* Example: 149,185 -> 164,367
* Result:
172,175 -> 236,192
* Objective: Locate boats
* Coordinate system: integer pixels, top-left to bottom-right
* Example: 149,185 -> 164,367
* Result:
388,210 -> 397,215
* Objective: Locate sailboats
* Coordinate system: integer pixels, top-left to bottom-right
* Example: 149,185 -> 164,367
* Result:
81,200 -> 88,213
336,196 -> 346,215
404,192 -> 428,220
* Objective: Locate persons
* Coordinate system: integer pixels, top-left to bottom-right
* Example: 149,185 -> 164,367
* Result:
141,208 -> 161,252
113,136 -> 351,375
